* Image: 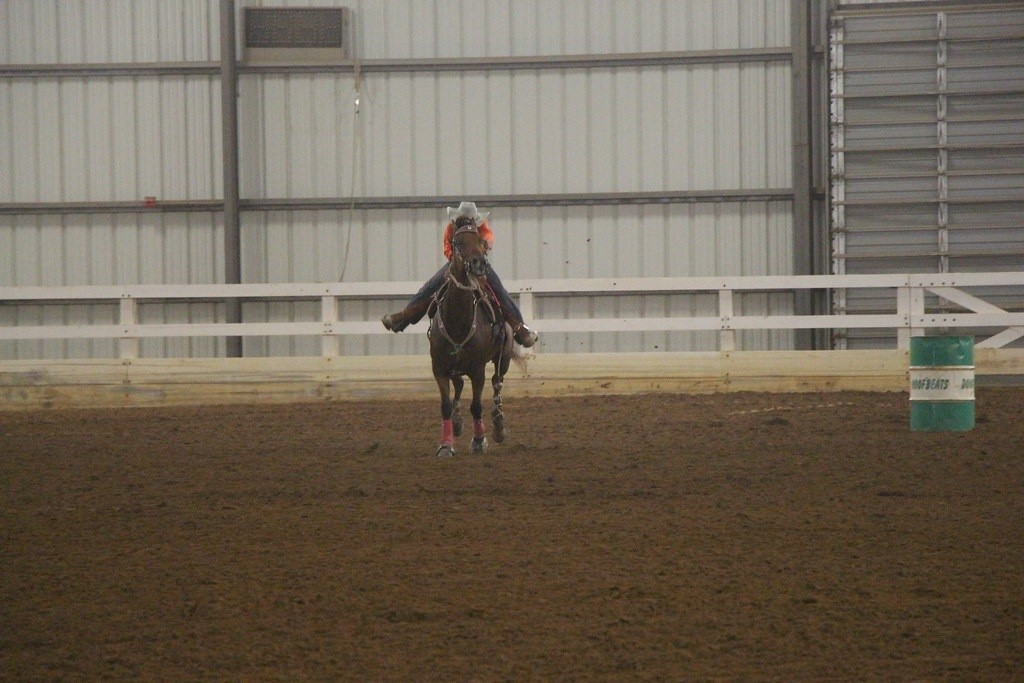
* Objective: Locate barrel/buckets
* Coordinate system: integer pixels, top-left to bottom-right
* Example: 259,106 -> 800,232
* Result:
910,335 -> 976,432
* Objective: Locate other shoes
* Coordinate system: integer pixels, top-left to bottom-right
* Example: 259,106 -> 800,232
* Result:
382,310 -> 409,330
513,322 -> 535,346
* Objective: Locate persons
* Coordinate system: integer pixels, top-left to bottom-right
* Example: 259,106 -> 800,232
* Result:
383,202 -> 534,347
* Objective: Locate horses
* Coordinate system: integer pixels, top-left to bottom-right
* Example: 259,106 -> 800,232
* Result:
429,216 -> 514,458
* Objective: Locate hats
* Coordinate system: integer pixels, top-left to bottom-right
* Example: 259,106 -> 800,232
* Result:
446,202 -> 490,228
